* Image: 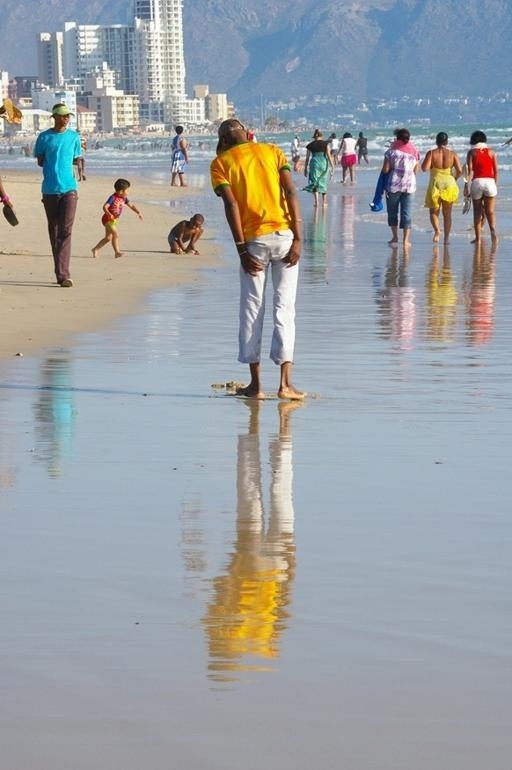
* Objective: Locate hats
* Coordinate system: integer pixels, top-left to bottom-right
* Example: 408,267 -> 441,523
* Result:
217,119 -> 246,156
52,105 -> 74,117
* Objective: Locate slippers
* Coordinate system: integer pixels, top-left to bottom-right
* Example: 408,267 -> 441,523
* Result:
3,204 -> 18,226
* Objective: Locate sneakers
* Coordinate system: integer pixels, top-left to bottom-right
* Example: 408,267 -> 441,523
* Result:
61,279 -> 74,287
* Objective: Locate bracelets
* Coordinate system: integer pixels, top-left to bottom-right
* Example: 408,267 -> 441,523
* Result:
291,217 -> 304,223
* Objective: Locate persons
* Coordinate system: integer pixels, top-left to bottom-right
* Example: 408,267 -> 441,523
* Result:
467,130 -> 499,244
167,214 -> 204,255
1,96 -> 24,227
291,130 -> 368,207
33,104 -> 86,287
420,132 -> 463,245
382,128 -> 421,245
203,398 -> 307,699
310,196 -> 497,351
211,117 -> 309,401
91,179 -> 144,259
35,346 -> 77,481
171,126 -> 188,187
